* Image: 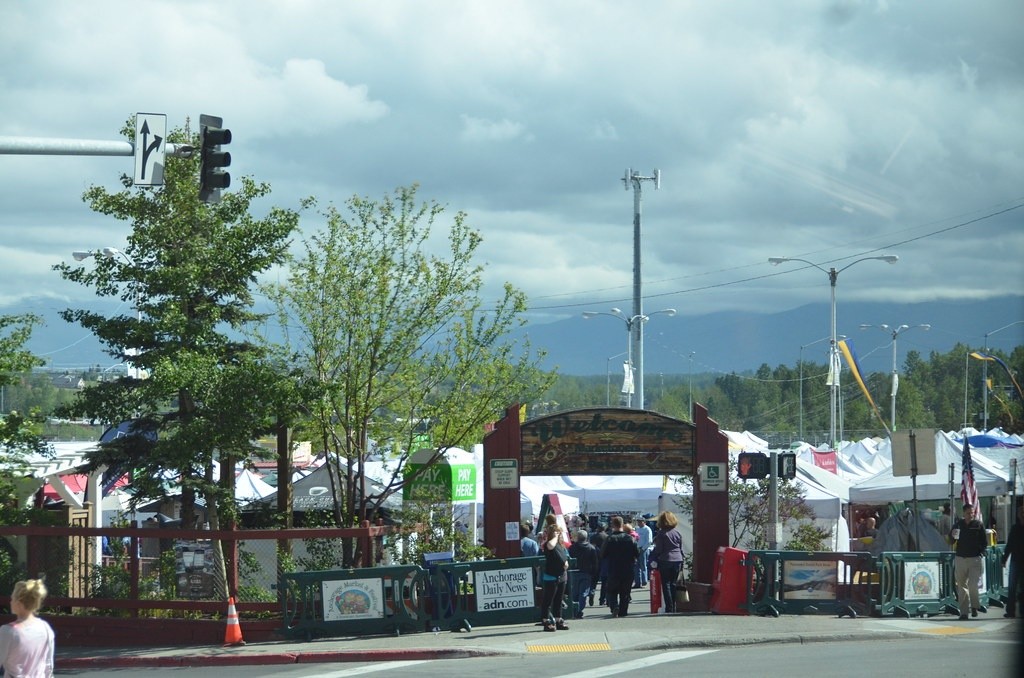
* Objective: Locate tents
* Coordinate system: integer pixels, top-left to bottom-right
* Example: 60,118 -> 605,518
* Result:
0,439 -> 693,603
722,427 -> 1024,552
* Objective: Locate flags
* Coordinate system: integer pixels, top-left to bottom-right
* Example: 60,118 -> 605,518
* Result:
962,438 -> 983,523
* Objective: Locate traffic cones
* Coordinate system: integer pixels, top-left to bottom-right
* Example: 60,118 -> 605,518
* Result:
222,598 -> 247,648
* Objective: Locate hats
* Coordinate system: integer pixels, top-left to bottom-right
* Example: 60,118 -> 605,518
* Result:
637,517 -> 644,521
597,522 -> 606,528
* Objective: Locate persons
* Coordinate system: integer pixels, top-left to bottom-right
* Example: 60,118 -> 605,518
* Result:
1002,505 -> 1024,618
519,511 -> 684,632
866,518 -> 878,538
856,518 -> 865,538
948,505 -> 988,620
942,503 -> 950,516
0,578 -> 55,678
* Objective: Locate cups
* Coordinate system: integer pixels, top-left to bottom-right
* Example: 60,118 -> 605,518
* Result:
954,529 -> 960,539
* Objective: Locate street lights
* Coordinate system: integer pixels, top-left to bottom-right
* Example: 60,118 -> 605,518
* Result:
582,309 -> 678,409
689,350 -> 698,420
769,256 -> 897,447
860,323 -> 930,433
800,335 -> 847,440
777,452 -> 797,481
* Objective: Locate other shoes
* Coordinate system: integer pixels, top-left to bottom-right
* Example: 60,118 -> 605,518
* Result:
590,600 -> 593,606
543,618 -> 556,631
629,597 -> 632,603
665,602 -> 673,612
554,617 -> 568,630
612,605 -> 620,618
632,584 -> 640,588
620,610 -> 627,616
972,611 -> 977,617
642,582 -> 647,586
600,599 -> 604,605
1004,613 -> 1015,618
579,610 -> 583,619
959,615 -> 968,620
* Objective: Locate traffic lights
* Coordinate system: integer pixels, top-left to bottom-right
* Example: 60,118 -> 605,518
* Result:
737,451 -> 767,479
198,113 -> 231,201
